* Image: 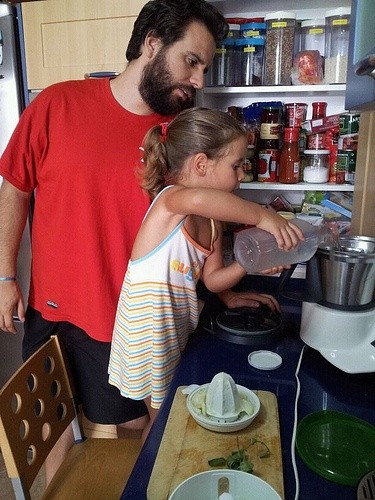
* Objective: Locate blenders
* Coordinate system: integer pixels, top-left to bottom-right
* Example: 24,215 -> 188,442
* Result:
278,235 -> 375,373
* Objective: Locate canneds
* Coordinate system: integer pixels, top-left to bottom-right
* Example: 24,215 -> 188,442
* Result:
228,102 -> 360,184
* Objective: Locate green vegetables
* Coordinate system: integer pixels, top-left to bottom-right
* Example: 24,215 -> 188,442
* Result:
208,434 -> 272,473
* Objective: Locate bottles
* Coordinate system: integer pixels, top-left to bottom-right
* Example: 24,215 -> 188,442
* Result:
211,6 -> 351,85
234,219 -> 334,271
279,102 -> 330,189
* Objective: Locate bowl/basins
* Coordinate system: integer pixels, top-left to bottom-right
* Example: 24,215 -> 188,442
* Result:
187,383 -> 260,432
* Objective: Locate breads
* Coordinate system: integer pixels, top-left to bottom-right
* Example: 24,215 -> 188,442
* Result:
294,51 -> 322,85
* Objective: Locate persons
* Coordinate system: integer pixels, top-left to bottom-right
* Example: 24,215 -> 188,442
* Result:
0,0 -> 281,486
107,106 -> 304,442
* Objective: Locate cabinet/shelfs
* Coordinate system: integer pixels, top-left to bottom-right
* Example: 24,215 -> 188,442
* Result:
194,0 -> 361,279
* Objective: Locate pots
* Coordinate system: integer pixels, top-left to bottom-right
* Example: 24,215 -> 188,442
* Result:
169,468 -> 282,500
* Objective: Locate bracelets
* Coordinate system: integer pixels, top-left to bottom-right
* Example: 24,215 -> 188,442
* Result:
0,278 -> 16,281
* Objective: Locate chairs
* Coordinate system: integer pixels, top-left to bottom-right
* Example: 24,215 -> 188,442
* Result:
0,333 -> 146,500
